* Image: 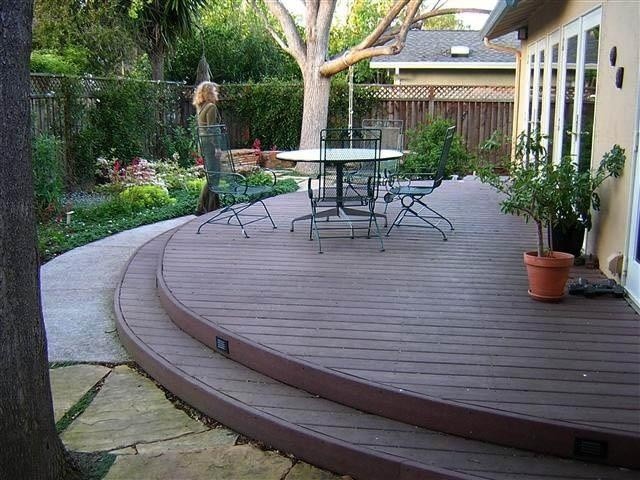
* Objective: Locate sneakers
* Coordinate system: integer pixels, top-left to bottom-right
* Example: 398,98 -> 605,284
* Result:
193,210 -> 204,215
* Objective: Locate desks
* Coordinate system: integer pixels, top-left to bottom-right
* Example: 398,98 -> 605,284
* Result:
276,148 -> 403,241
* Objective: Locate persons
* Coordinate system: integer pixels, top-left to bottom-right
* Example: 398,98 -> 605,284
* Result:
192,81 -> 224,216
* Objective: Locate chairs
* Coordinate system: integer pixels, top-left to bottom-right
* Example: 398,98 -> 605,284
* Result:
196,124 -> 277,239
384,125 -> 458,241
343,119 -> 404,214
290,128 -> 385,254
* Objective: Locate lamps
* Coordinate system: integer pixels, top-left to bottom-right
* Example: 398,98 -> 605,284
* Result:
518,27 -> 529,40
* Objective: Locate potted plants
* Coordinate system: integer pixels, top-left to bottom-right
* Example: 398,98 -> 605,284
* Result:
541,133 -> 594,259
473,122 -> 626,303
